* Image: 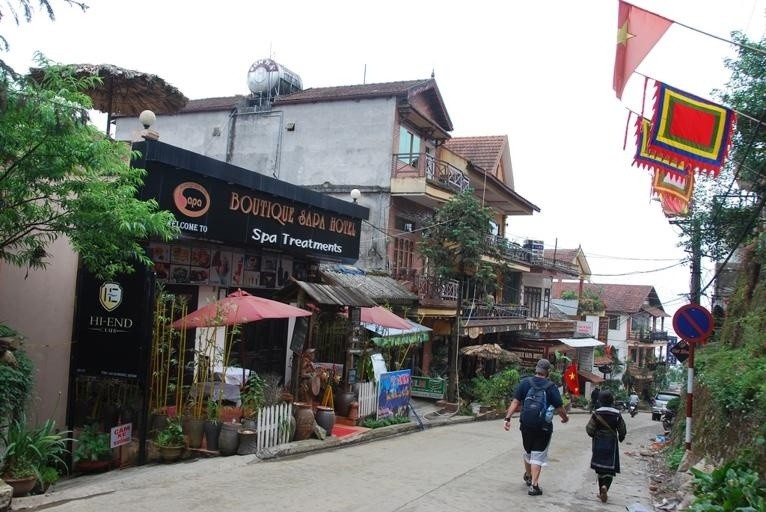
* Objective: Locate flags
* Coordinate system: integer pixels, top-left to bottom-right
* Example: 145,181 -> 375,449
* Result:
631,80 -> 736,217
613,0 -> 674,100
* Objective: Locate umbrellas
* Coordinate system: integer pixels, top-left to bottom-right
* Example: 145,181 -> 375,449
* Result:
307,303 -> 415,330
167,287 -> 313,389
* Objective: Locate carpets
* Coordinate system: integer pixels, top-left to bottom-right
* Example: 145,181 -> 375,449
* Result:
329,423 -> 356,438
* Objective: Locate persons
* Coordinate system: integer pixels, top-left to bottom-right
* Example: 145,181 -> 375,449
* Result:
589,388 -> 599,414
628,391 -> 640,412
503,358 -> 569,496
586,391 -> 626,503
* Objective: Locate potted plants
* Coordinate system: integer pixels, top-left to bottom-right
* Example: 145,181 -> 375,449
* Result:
149,279 -> 296,464
73,422 -> 111,475
0,398 -> 79,498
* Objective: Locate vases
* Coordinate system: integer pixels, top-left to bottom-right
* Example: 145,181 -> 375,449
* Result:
315,406 -> 334,436
292,402 -> 314,441
336,389 -> 356,416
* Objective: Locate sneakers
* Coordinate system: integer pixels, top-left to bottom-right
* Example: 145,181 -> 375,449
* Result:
596,484 -> 609,503
523,471 -> 533,487
527,484 -> 544,496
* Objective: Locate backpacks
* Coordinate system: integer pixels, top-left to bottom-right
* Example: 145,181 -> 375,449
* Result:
518,377 -> 557,430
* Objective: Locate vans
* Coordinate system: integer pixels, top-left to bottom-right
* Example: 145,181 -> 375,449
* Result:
649,392 -> 681,422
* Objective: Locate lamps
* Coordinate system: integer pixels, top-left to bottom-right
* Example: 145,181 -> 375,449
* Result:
350,188 -> 361,205
138,109 -> 156,141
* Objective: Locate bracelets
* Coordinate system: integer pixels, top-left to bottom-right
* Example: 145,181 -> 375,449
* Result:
505,418 -> 510,422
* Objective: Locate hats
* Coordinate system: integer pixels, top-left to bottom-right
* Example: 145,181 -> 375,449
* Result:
535,358 -> 552,377
597,390 -> 615,405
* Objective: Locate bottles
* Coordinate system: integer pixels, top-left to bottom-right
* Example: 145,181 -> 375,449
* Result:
545,405 -> 555,422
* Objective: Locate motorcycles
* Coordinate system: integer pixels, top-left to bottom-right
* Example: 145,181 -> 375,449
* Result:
613,400 -> 627,412
659,409 -> 676,432
629,405 -> 637,418
